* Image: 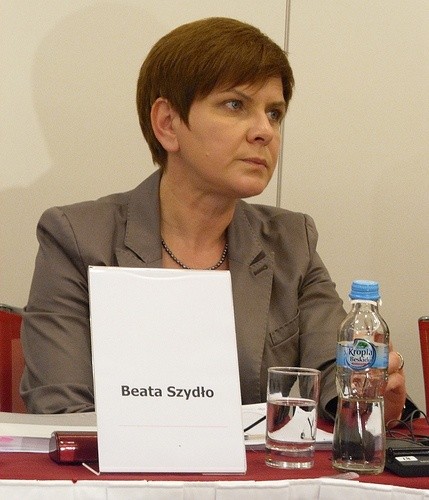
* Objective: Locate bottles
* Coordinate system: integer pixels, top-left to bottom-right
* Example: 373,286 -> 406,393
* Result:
331,280 -> 390,475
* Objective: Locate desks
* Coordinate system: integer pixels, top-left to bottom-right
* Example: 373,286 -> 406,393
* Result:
0,417 -> 429,500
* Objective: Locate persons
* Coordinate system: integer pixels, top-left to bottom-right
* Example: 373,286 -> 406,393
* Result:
23,16 -> 407,428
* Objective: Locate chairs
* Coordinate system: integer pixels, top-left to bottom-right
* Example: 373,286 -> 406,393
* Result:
0,303 -> 30,414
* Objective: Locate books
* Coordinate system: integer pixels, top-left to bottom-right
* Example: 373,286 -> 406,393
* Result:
0,409 -> 98,454
241,402 -> 334,451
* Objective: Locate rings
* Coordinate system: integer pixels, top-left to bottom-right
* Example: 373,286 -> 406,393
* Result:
395,352 -> 404,371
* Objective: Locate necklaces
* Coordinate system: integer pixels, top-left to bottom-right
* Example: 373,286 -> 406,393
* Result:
156,235 -> 229,270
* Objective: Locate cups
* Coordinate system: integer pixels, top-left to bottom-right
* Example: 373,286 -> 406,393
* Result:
264,367 -> 320,470
418,316 -> 429,418
